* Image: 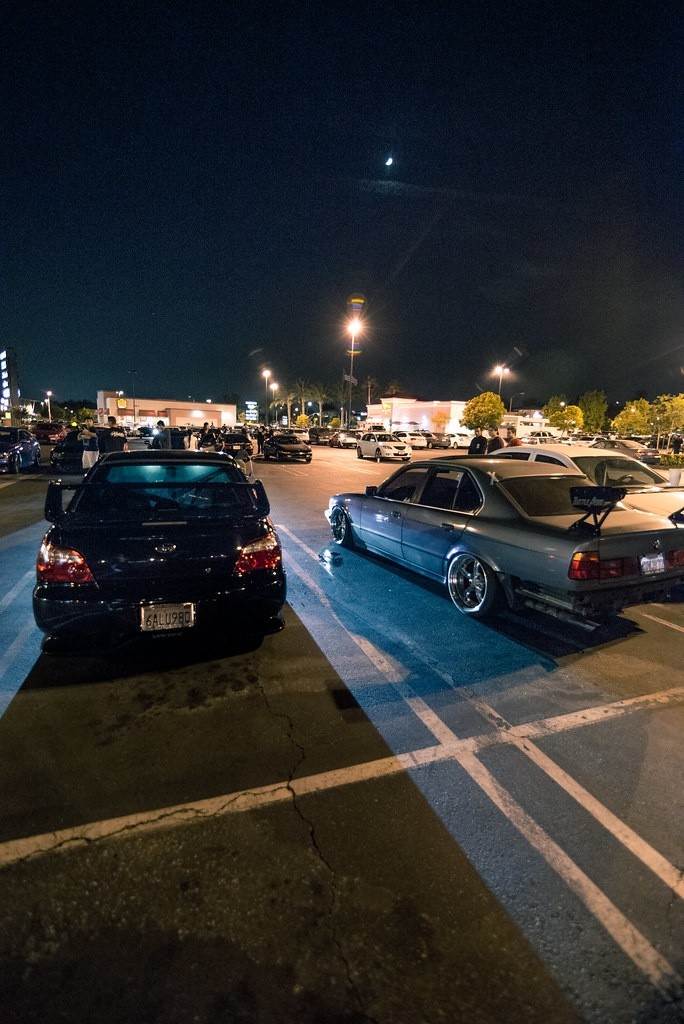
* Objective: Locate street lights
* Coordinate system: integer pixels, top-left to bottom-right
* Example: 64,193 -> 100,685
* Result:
347,322 -> 358,434
509,392 -> 525,412
270,383 -> 277,429
127,370 -> 139,440
498,367 -> 509,395
263,370 -> 271,427
47,391 -> 52,423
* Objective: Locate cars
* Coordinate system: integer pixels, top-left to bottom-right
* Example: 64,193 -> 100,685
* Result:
356,431 -> 413,461
323,455 -> 684,635
50,427 -> 110,474
308,426 -> 683,451
143,430 -> 188,450
486,444 -> 684,521
587,440 -> 661,464
198,433 -> 254,458
262,434 -> 312,462
30,423 -> 67,445
32,450 -> 287,658
119,425 -> 310,444
0,426 -> 43,474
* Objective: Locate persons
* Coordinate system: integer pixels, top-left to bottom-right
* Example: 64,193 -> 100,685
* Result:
156,420 -> 172,449
257,427 -> 264,454
486,425 -> 505,454
98,416 -> 128,454
198,422 -> 216,447
210,422 -> 215,429
673,435 -> 683,454
77,417 -> 99,473
468,426 -> 487,454
233,444 -> 257,478
506,426 -> 523,447
242,423 -> 247,437
222,424 -> 228,433
183,429 -> 198,449
